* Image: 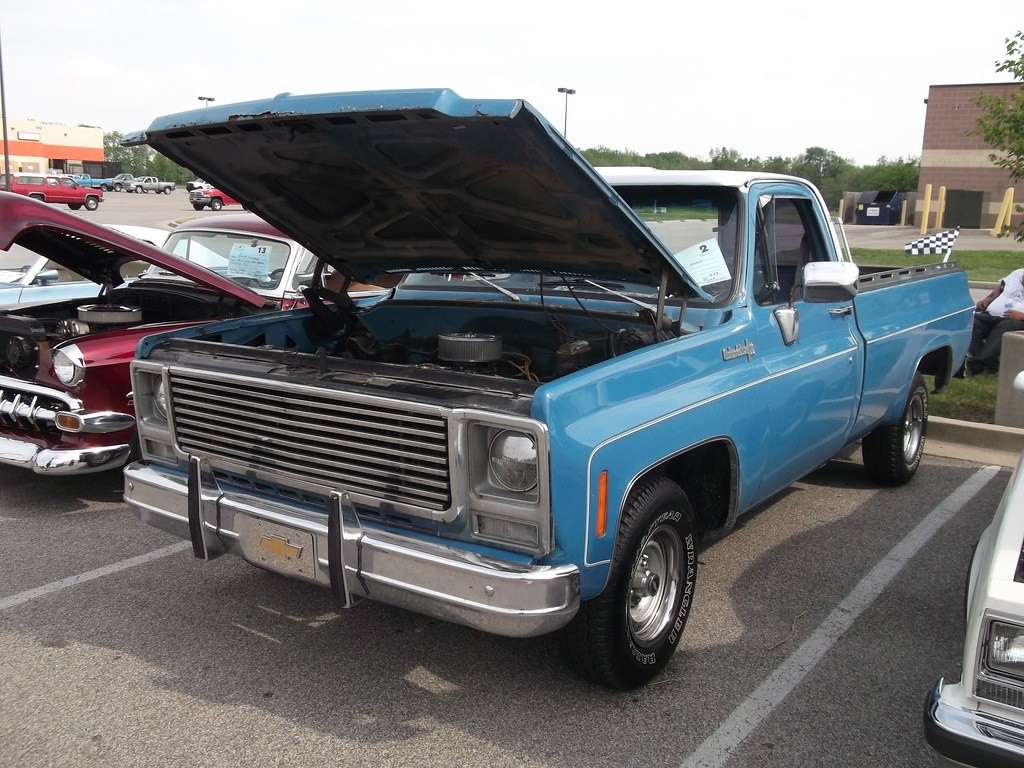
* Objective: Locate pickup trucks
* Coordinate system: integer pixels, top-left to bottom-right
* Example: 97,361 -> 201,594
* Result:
74,174 -> 114,192
186,178 -> 215,193
113,173 -> 136,193
124,176 -> 175,195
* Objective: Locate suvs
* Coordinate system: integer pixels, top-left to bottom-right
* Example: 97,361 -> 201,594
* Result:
189,187 -> 248,211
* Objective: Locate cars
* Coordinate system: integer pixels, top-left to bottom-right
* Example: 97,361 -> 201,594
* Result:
0,173 -> 105,211
123,87 -> 977,689
924,365 -> 1024,768
0,189 -> 509,475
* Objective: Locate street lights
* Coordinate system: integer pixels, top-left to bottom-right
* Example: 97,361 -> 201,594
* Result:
558,88 -> 575,139
198,97 -> 215,108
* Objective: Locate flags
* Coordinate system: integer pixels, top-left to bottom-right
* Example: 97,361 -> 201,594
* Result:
905,227 -> 960,255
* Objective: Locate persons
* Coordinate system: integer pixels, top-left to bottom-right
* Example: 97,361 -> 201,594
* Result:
967,268 -> 1024,374
